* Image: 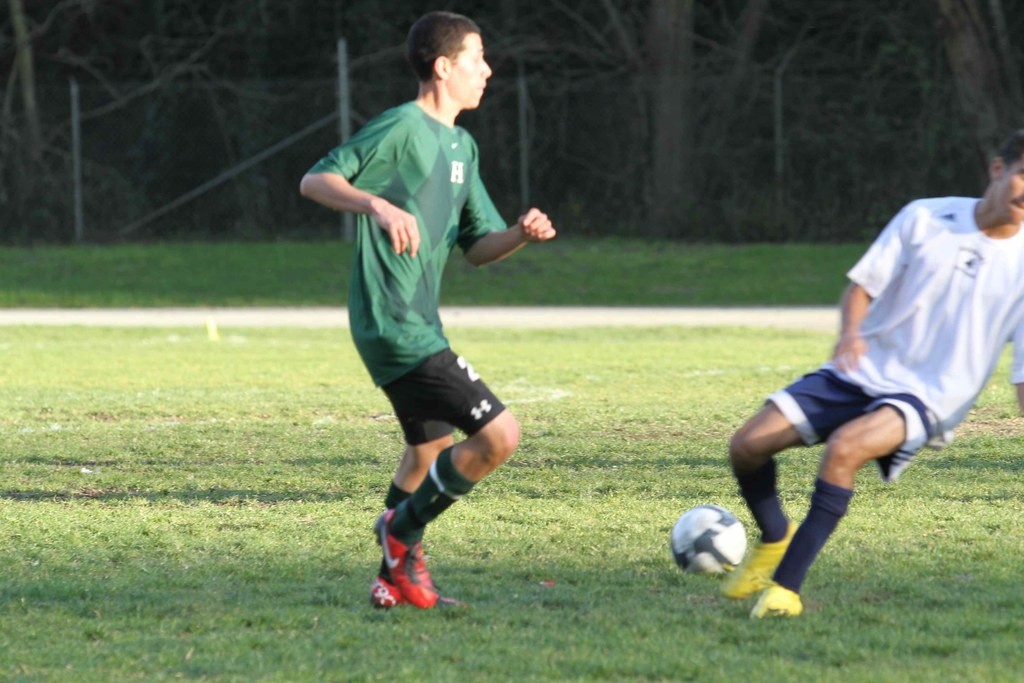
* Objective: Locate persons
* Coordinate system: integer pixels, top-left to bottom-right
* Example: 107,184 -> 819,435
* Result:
299,11 -> 557,610
716,127 -> 1024,623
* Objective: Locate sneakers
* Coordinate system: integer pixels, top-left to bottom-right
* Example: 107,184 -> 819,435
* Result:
721,519 -> 797,600
370,557 -> 402,609
750,568 -> 802,620
373,509 -> 437,607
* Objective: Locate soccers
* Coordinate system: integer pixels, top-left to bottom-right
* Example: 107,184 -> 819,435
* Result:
669,504 -> 748,577
